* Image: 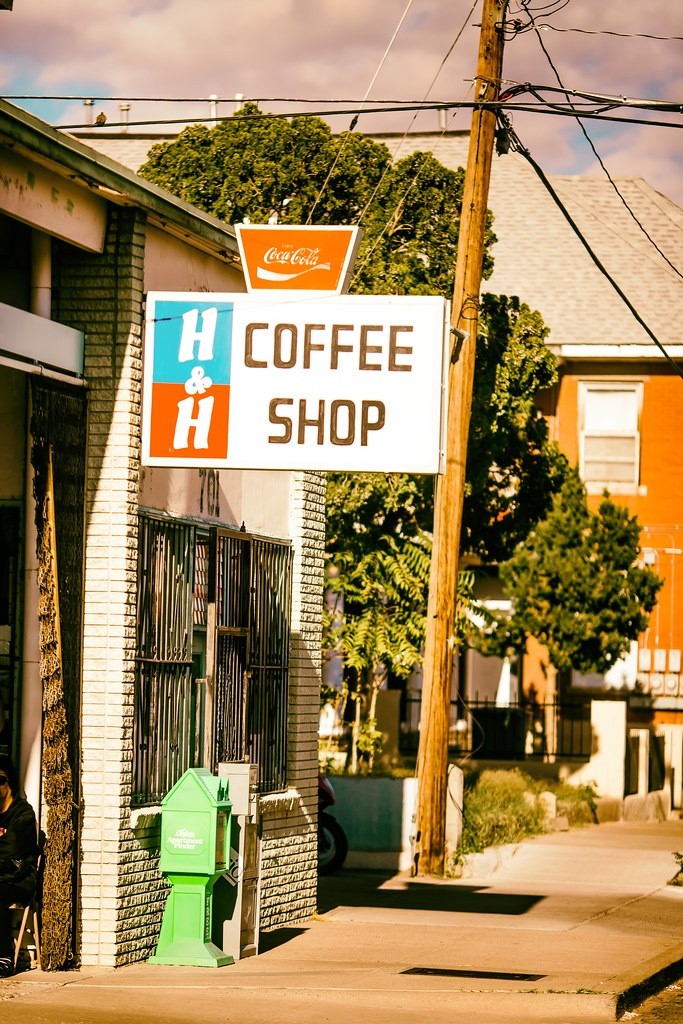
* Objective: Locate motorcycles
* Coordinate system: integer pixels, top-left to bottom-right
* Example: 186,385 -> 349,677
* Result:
317,772 -> 349,877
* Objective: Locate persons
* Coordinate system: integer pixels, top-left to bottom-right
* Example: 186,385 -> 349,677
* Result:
0,755 -> 36,977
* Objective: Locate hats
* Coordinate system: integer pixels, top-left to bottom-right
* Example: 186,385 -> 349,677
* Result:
0,754 -> 14,775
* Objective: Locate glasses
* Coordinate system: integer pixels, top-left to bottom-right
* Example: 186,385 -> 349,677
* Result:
0,775 -> 9,786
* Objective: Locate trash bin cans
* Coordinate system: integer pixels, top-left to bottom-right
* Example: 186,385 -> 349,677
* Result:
470,707 -> 534,762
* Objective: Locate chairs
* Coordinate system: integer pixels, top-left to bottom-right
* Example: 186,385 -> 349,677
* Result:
8,855 -> 42,974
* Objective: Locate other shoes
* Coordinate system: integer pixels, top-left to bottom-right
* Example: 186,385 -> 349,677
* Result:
0,957 -> 14,977
